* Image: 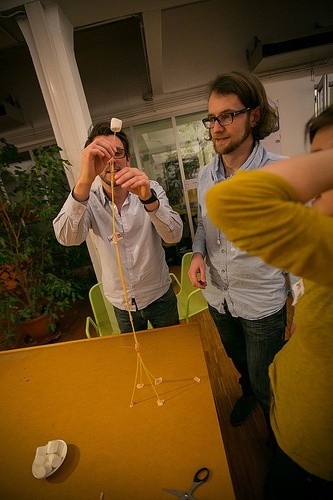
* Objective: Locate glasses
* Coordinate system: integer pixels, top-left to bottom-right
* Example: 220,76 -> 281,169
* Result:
113,149 -> 127,159
202,107 -> 250,129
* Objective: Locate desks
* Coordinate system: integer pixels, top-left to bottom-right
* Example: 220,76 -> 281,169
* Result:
0,322 -> 238,500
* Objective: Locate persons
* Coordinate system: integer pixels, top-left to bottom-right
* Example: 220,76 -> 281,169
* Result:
52,121 -> 183,334
205,104 -> 333,500
187,71 -> 302,448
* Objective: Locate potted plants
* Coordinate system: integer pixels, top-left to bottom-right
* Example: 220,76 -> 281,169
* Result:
0,144 -> 84,349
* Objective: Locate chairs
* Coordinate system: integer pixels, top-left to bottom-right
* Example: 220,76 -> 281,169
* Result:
169,252 -> 209,324
85,282 -> 154,339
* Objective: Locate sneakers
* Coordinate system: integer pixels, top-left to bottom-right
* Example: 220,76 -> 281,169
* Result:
230,395 -> 257,426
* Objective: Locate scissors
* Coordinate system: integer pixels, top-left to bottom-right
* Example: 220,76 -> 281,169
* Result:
161,466 -> 211,500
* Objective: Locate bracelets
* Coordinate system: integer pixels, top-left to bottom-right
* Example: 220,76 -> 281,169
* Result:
139,188 -> 158,204
